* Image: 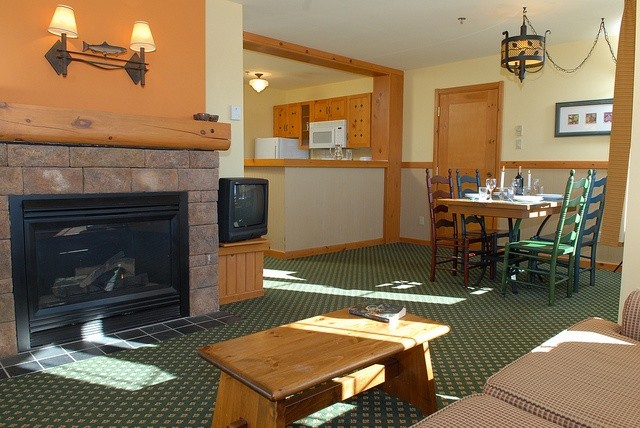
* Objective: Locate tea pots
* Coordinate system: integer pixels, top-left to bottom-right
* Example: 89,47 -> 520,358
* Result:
329,144 -> 343,160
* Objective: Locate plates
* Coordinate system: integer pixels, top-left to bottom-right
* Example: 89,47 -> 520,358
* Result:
539,193 -> 564,200
514,193 -> 542,202
465,192 -> 490,200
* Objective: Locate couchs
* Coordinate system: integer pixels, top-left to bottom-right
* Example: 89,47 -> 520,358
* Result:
408,290 -> 640,428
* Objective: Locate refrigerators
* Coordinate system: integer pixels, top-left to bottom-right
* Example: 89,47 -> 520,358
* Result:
254,136 -> 310,160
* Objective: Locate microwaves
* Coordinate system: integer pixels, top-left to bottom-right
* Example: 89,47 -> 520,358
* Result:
309,119 -> 347,149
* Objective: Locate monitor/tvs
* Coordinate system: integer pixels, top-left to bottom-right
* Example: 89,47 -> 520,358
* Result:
216,177 -> 269,243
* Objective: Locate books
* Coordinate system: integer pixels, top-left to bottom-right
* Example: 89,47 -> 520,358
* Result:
350,300 -> 407,325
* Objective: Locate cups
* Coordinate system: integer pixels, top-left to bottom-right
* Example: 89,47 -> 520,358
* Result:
478,186 -> 488,201
346,149 -> 352,160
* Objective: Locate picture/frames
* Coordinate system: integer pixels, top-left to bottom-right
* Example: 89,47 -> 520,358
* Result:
553,98 -> 615,139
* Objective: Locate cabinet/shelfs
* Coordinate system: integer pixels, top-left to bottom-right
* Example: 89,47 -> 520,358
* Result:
299,99 -> 309,149
348,93 -> 371,150
312,96 -> 347,122
273,102 -> 301,138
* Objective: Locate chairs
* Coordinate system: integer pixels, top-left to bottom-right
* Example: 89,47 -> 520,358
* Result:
573,170 -> 607,292
455,169 -> 521,295
499,169 -> 594,307
424,168 -> 500,288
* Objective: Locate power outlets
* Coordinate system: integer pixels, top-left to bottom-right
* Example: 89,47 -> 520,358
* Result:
515,139 -> 523,150
230,104 -> 241,120
514,125 -> 523,137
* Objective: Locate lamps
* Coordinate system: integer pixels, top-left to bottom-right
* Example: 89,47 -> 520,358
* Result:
248,73 -> 269,94
45,2 -> 156,90
501,6 -> 551,84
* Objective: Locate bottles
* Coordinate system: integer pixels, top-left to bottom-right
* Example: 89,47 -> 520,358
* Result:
514,165 -> 524,197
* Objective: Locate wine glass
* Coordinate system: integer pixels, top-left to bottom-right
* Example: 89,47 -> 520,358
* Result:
486,178 -> 496,202
511,178 -> 521,195
532,178 -> 541,196
